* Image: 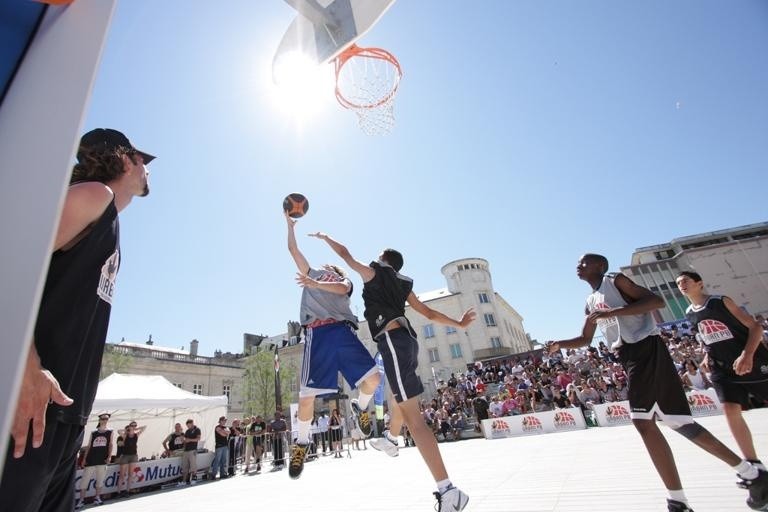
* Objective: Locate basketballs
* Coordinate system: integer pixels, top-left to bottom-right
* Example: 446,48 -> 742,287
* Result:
283,192 -> 308,218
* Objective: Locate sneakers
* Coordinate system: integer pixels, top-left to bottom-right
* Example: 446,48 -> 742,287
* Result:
334,454 -> 342,458
245,467 -> 249,473
667,499 -> 691,512
210,474 -> 231,481
737,458 -> 767,511
255,458 -> 261,471
370,435 -> 400,457
94,498 -> 103,505
434,487 -> 470,511
353,399 -> 373,436
289,438 -> 310,476
115,491 -> 129,497
75,500 -> 85,508
178,479 -> 196,486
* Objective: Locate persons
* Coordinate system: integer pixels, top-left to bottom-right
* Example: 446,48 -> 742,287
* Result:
420,340 -> 628,442
755,314 -> 768,346
0,127 -> 158,511
384,409 -> 415,446
544,254 -> 768,512
284,210 -> 381,480
307,232 -> 478,511
675,271 -> 768,488
660,323 -> 711,392
72,409 -> 368,511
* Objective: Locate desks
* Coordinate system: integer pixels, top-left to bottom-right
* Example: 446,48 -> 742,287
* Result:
592,389 -> 723,427
74,451 -> 215,500
481,407 -> 588,439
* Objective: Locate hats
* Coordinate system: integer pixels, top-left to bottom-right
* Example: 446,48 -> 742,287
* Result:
77,126 -> 157,165
